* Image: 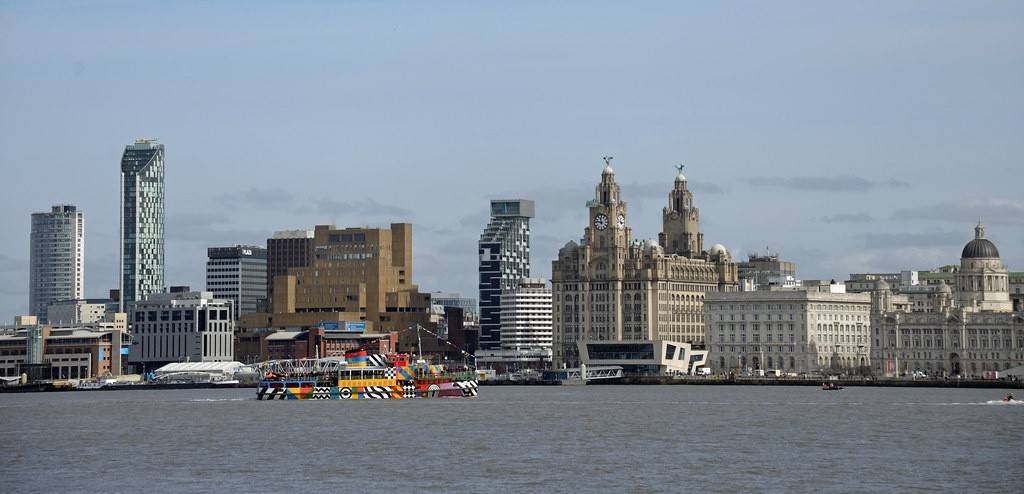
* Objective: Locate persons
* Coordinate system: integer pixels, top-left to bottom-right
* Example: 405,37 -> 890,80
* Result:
265,369 -> 338,380
439,363 -> 476,374
397,376 -> 418,386
353,370 -> 385,379
823,383 -> 839,389
999,372 -> 1018,382
1004,393 -> 1014,402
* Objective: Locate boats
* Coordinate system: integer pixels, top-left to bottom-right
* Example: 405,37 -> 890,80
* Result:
256,324 -> 479,401
822,385 -> 844,391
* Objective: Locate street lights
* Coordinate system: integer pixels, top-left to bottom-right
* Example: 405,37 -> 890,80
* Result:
178,355 -> 184,374
884,314 -> 899,379
